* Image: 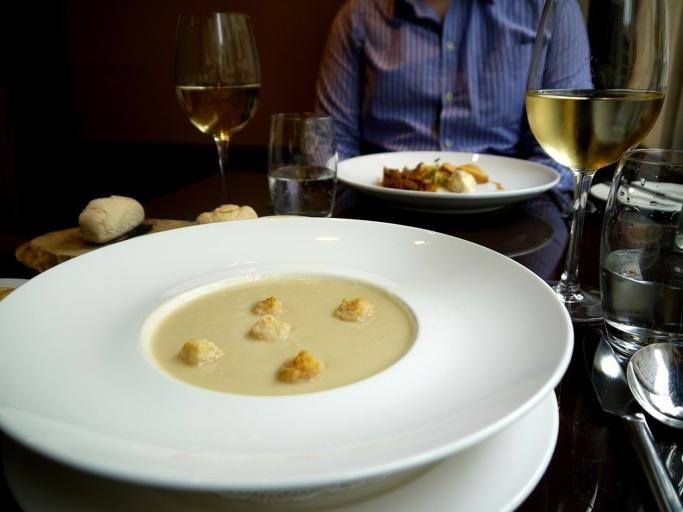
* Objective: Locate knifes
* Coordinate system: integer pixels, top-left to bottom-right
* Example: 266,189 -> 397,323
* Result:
588,335 -> 683,512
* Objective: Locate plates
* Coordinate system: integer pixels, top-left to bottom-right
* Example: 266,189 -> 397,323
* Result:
331,147 -> 562,217
1,212 -> 580,512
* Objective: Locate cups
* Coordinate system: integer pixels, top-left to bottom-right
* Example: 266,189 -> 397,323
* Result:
264,110 -> 340,218
594,144 -> 683,359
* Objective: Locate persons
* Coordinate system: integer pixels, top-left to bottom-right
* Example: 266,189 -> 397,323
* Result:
302,0 -> 602,198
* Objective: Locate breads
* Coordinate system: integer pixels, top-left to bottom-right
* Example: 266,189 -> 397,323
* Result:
195,204 -> 258,225
79,194 -> 145,244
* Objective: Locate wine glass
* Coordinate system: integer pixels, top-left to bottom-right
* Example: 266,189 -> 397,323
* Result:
524,86 -> 667,324
172,9 -> 260,203
